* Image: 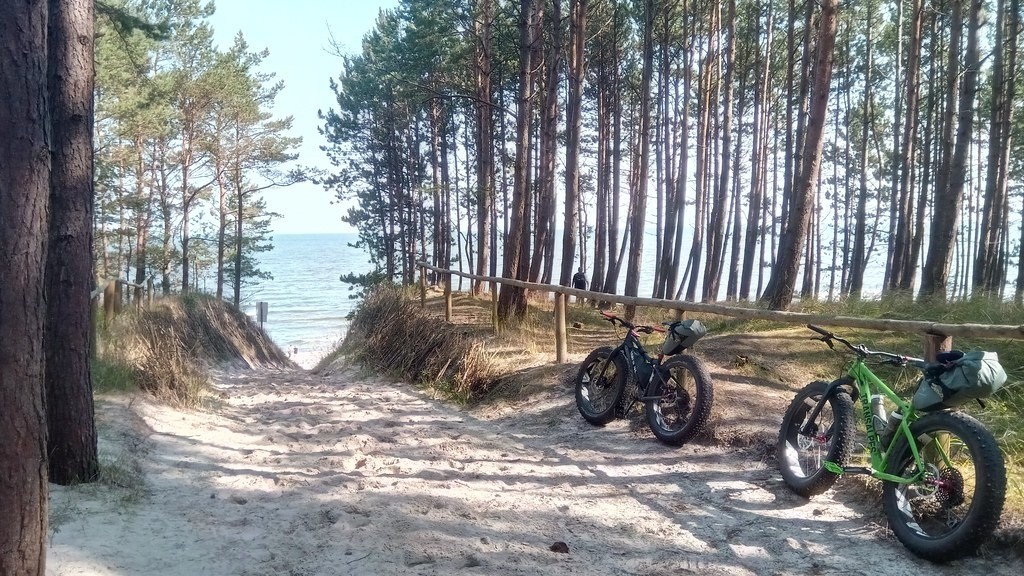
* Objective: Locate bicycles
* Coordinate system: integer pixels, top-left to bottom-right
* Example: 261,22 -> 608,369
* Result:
776,321 -> 1008,563
575,310 -> 714,447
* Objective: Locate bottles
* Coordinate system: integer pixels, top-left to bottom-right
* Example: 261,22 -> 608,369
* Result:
881,406 -> 903,446
871,394 -> 887,435
638,363 -> 652,388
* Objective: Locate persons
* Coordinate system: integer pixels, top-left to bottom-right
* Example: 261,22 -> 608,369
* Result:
572,266 -> 589,306
